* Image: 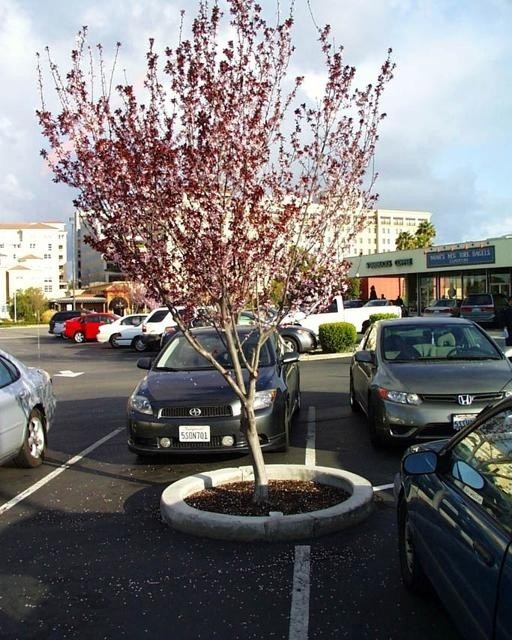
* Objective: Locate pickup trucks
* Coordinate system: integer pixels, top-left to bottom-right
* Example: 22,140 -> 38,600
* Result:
278,293 -> 403,354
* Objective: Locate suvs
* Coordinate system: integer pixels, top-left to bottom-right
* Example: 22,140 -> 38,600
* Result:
142,306 -> 220,350
49,311 -> 94,340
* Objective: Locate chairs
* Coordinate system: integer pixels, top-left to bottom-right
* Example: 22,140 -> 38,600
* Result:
430,331 -> 459,357
384,334 -> 413,359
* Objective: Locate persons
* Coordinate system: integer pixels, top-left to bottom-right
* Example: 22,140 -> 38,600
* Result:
395,296 -> 403,306
369,285 -> 377,300
382,294 -> 385,299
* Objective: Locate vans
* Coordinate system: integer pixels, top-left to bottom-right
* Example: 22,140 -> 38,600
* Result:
459,293 -> 508,328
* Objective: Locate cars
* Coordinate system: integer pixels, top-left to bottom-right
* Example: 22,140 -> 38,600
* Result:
349,316 -> 512,446
423,299 -> 463,317
344,299 -> 367,308
0,349 -> 57,469
97,313 -> 148,348
235,308 -> 317,354
363,299 -> 406,316
127,326 -> 302,456
61,312 -> 122,342
389,395 -> 512,640
245,307 -> 285,321
114,321 -> 146,351
